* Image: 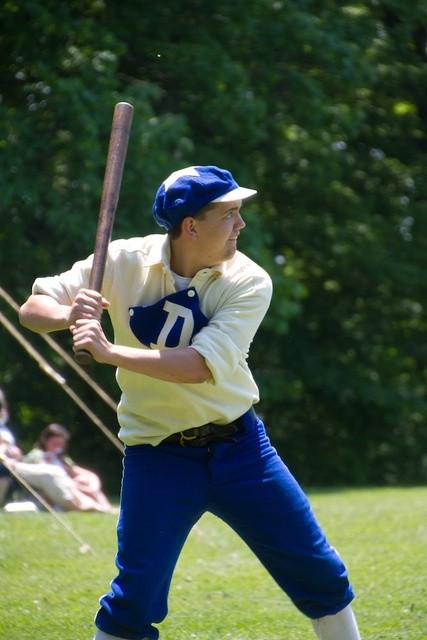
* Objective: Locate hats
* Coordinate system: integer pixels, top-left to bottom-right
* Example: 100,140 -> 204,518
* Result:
152,165 -> 258,232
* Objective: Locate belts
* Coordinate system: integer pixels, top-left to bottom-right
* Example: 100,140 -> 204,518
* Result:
165,426 -> 240,446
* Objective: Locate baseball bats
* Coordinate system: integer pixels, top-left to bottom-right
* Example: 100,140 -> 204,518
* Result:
74,101 -> 135,365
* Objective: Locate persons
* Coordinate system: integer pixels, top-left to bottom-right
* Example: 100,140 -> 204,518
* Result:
0,390 -> 92,511
19,163 -> 358,640
22,423 -> 118,514
